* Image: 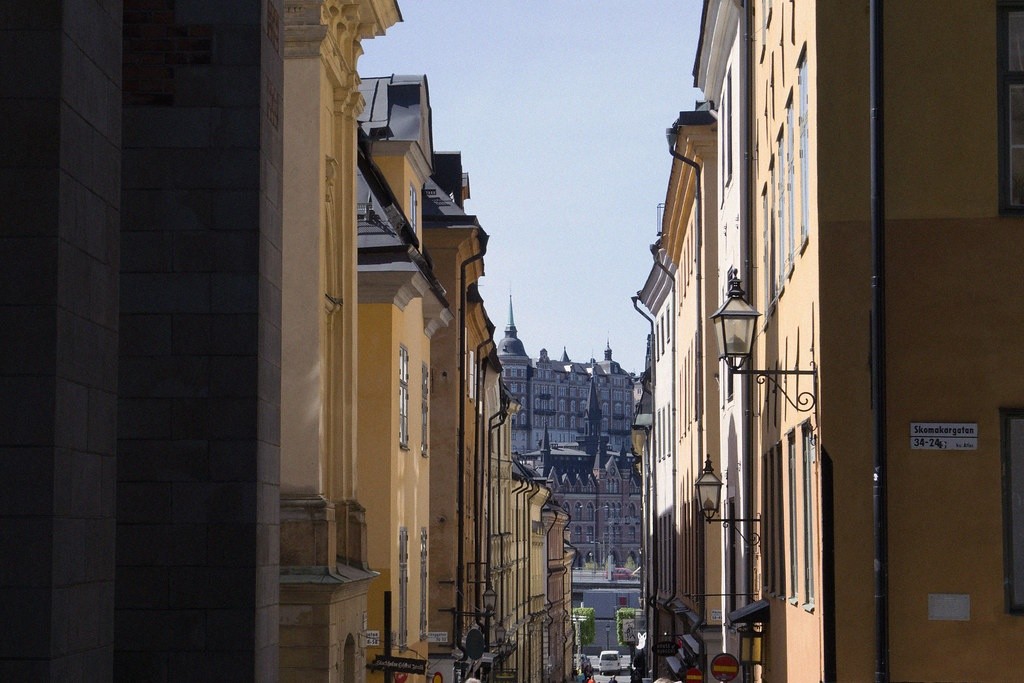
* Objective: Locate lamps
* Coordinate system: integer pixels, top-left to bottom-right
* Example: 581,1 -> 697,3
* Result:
489,398 -> 522,429
702,267 -> 819,431
692,454 -> 761,554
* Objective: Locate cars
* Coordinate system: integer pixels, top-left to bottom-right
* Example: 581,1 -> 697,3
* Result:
606,568 -> 637,581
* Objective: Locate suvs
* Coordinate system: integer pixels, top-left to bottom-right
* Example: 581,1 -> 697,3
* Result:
574,654 -> 592,673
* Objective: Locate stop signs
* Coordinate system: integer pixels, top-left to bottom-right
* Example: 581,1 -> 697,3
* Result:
685,668 -> 703,683
711,653 -> 739,683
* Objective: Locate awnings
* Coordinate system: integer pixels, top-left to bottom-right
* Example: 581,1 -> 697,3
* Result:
726,599 -> 770,624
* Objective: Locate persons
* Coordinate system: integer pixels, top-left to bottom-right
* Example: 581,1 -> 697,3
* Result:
581,655 -> 595,683
609,675 -> 617,683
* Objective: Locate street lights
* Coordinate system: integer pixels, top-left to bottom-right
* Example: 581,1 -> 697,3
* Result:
589,552 -> 593,574
606,623 -> 610,651
580,505 -> 610,574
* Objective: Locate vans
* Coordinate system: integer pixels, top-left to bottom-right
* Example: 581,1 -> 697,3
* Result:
598,650 -> 623,675
